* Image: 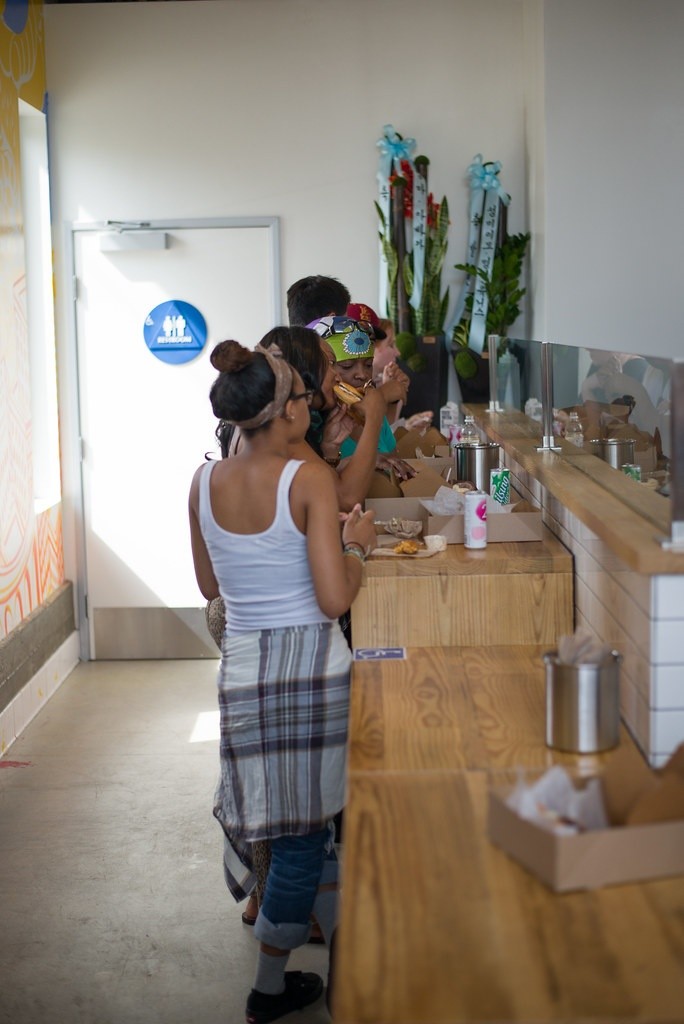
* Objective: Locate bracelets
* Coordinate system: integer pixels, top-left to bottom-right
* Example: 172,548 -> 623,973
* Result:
343,546 -> 365,564
323,450 -> 342,466
341,541 -> 367,558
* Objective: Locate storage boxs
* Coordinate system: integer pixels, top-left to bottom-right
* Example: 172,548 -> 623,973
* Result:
561,400 -> 657,473
364,425 -> 544,545
485,737 -> 684,894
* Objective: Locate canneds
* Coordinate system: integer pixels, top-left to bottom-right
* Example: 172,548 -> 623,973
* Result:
449,423 -> 462,455
619,463 -> 642,483
553,419 -> 565,438
463,490 -> 487,550
490,468 -> 510,505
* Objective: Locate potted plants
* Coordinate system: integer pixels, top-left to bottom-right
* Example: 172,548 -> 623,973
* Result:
449,214 -> 531,403
373,195 -> 449,432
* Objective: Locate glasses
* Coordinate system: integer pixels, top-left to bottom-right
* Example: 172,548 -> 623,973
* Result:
293,389 -> 313,406
320,320 -> 372,341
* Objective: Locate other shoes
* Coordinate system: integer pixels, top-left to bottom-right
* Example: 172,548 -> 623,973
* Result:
242,913 -> 255,925
246,970 -> 323,1023
307,936 -> 324,944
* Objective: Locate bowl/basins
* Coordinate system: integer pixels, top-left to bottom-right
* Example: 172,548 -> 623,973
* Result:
424,535 -> 448,552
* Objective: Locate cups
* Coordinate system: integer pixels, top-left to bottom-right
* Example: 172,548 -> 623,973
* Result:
542,648 -> 625,755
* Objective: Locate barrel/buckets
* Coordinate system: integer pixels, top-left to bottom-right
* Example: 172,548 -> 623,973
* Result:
590,438 -> 637,472
453,442 -> 501,494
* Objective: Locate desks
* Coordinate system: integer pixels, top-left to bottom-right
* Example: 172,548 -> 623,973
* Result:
330,643 -> 684,1024
350,485 -> 574,653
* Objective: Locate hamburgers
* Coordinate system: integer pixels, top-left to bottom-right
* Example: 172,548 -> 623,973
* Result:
333,382 -> 363,405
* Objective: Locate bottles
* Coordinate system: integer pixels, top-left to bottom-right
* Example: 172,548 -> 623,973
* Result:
565,411 -> 584,449
494,348 -> 521,414
461,416 -> 480,444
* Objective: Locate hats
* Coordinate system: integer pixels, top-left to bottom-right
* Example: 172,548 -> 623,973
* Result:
347,303 -> 387,340
305,316 -> 374,362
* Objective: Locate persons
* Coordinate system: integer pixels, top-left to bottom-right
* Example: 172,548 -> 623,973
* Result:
579,347 -> 675,492
187,275 -> 410,1019
